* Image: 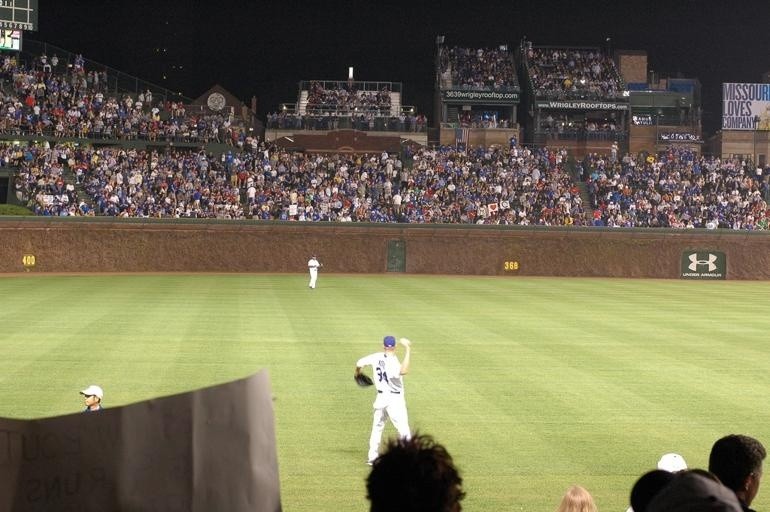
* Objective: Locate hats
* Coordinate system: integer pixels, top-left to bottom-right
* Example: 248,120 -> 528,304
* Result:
79,385 -> 103,400
384,336 -> 395,347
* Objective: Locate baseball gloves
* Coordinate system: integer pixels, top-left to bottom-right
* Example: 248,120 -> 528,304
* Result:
356,374 -> 373,386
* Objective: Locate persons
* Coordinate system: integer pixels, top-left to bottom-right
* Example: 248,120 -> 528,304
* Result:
626,453 -> 687,512
307,255 -> 320,289
709,434 -> 766,512
557,485 -> 597,512
366,428 -> 467,512
355,336 -> 413,463
80,385 -> 103,412
1,42 -> 769,232
